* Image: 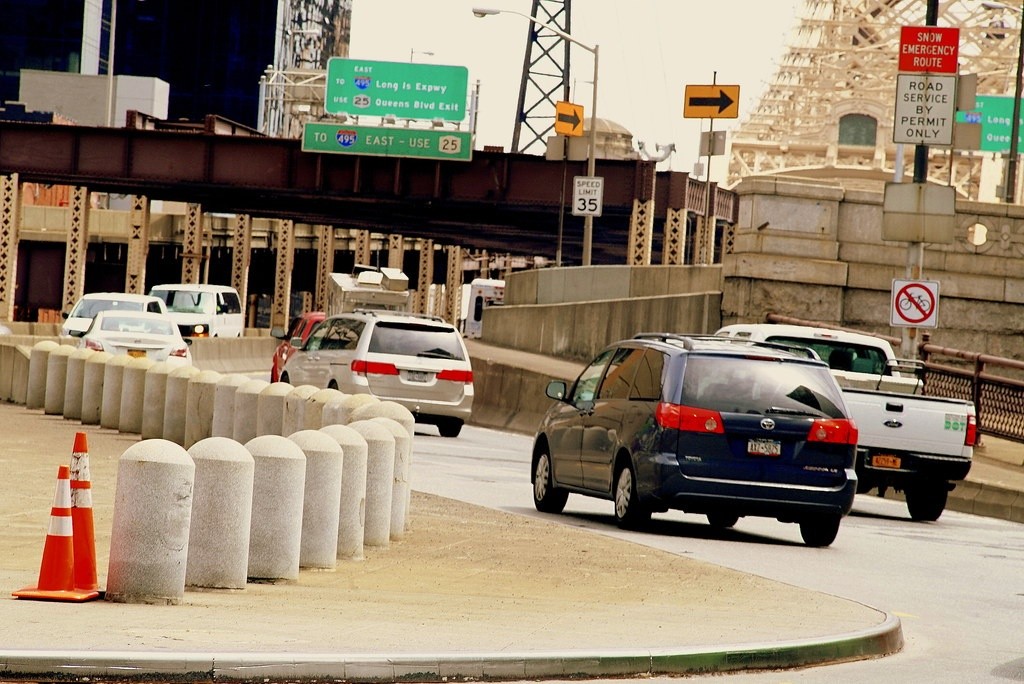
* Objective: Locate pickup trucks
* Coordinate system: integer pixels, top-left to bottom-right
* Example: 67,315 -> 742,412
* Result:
713,324 -> 976,522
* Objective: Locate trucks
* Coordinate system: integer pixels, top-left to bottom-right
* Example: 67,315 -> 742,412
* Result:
465,278 -> 506,338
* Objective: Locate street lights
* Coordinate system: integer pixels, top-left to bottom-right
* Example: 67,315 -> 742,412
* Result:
409,48 -> 434,62
471,6 -> 599,266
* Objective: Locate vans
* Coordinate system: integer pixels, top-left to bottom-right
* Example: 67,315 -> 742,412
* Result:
59,292 -> 168,339
277,309 -> 476,439
530,332 -> 859,548
147,283 -> 243,348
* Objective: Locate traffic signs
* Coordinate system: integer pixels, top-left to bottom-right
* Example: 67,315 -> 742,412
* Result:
323,58 -> 469,123
892,26 -> 961,146
302,123 -> 473,161
955,93 -> 1024,154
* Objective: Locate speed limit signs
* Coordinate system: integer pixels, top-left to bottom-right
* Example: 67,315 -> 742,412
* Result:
572,176 -> 604,217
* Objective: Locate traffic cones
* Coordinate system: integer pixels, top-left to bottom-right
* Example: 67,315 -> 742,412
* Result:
11,465 -> 100,602
68,431 -> 107,594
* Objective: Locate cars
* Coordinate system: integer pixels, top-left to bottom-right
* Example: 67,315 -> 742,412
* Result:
269,313 -> 327,385
68,310 -> 193,367
